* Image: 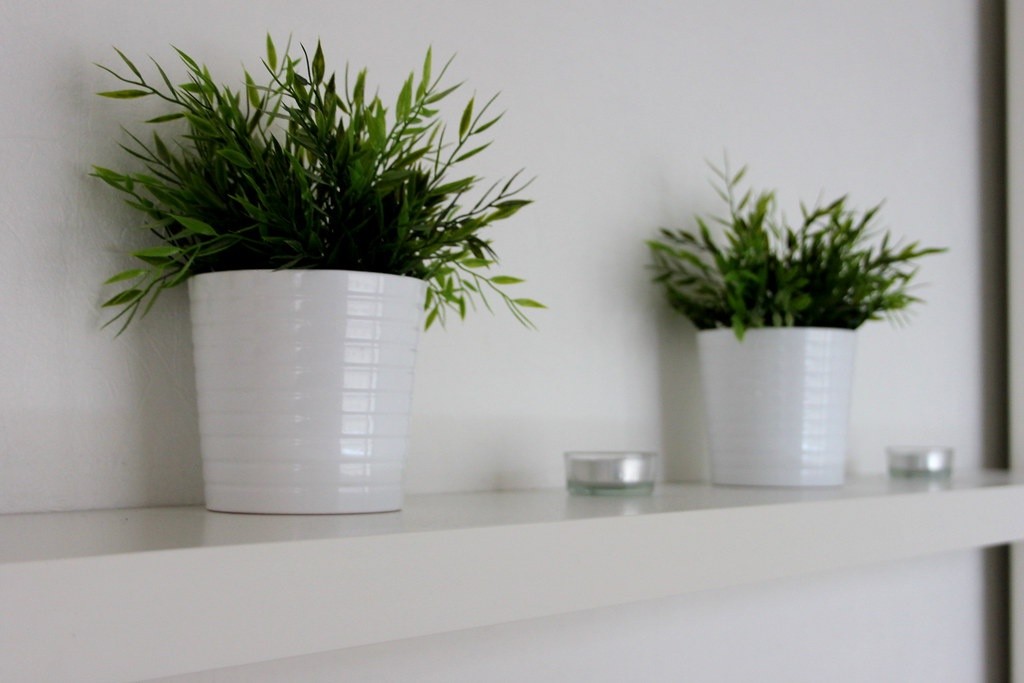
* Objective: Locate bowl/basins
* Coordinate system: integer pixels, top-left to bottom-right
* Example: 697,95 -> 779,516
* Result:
886,447 -> 953,479
564,451 -> 656,496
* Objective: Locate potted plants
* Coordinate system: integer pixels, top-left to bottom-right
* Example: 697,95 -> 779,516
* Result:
83,17 -> 543,517
641,148 -> 950,492
